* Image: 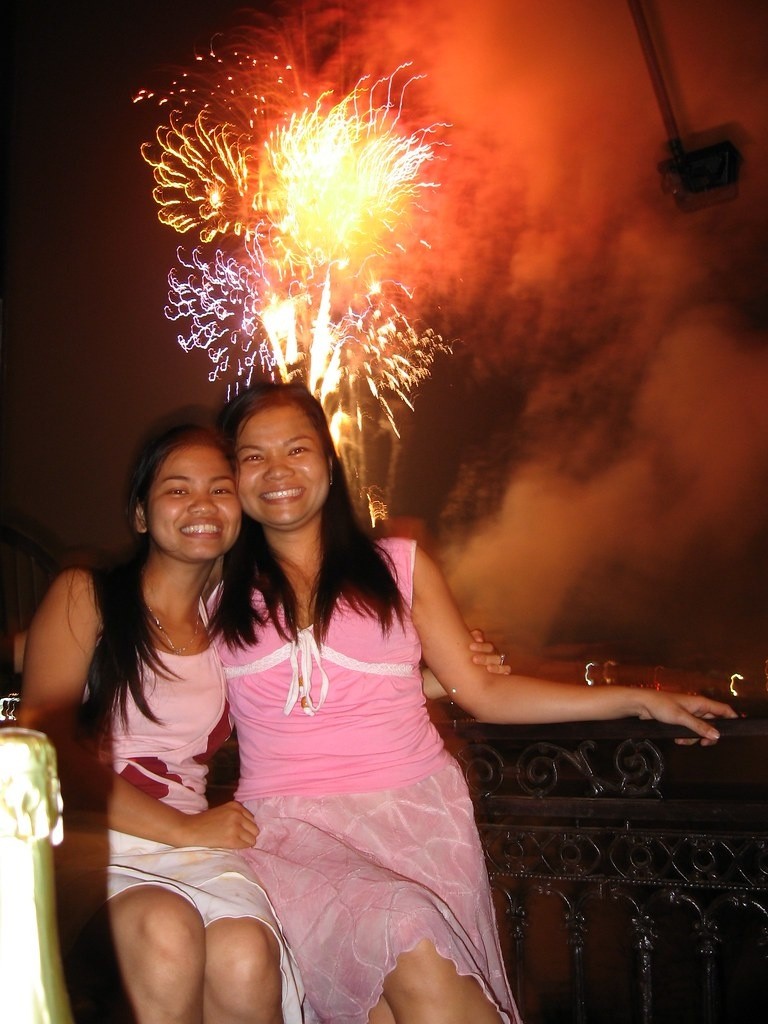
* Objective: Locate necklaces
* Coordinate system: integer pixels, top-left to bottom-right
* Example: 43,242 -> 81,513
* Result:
145,604 -> 200,655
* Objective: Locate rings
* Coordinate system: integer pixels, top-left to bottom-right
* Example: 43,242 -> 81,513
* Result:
501,652 -> 506,665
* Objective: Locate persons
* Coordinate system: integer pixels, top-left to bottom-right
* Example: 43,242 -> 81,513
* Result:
18,411 -> 510,1024
201,382 -> 737,1024
